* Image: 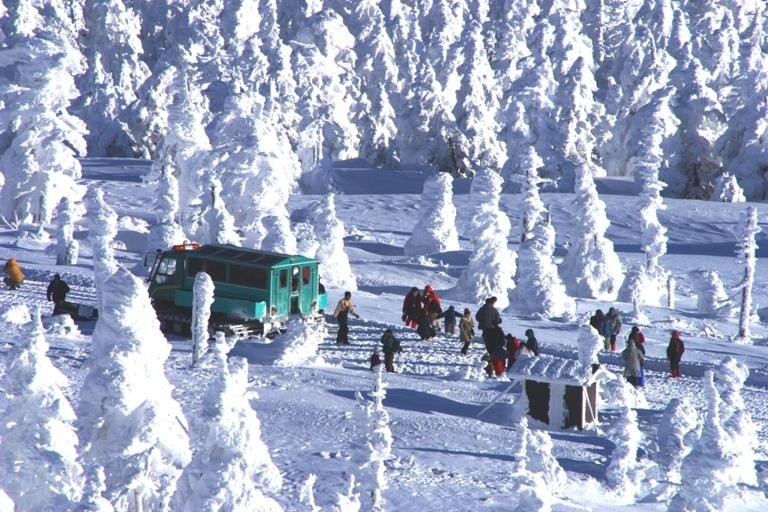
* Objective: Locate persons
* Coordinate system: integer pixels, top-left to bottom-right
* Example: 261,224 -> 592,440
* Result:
2,258 -> 24,290
603,307 -> 622,351
330,290 -> 361,346
401,286 -> 539,376
45,273 -> 70,304
634,340 -> 646,388
622,339 -> 643,386
665,329 -> 684,379
626,326 -> 648,343
588,309 -> 610,352
380,329 -> 398,372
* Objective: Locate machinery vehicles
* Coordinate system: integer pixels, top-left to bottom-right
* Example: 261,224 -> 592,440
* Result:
138,238 -> 332,342
